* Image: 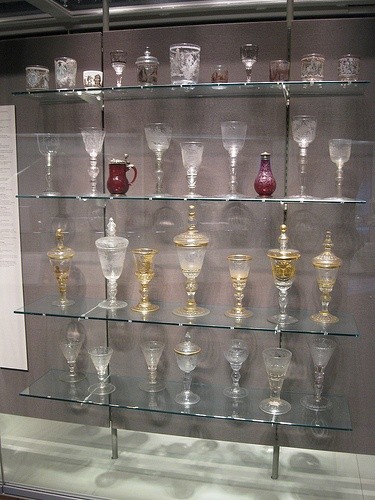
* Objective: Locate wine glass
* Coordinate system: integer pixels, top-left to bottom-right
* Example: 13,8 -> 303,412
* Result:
287,114 -> 320,200
225,254 -> 255,319
79,126 -> 106,196
218,121 -> 248,197
311,231 -> 343,324
174,206 -> 211,317
258,347 -> 293,414
88,346 -> 116,395
172,331 -> 202,404
222,336 -> 249,398
324,139 -> 354,200
131,248 -> 159,312
143,122 -> 174,198
108,49 -> 128,88
138,341 -> 168,392
59,340 -> 86,383
240,43 -> 257,83
266,224 -> 301,324
36,133 -> 61,196
95,217 -> 129,309
46,228 -> 74,307
301,338 -> 338,411
178,142 -> 206,198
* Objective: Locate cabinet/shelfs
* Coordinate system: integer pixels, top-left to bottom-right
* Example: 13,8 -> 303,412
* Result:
11,79 -> 370,433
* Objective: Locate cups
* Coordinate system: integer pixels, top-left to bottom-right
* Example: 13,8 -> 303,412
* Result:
107,153 -> 138,197
25,43 -> 359,90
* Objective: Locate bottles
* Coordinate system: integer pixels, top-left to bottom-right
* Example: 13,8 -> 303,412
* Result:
254,151 -> 276,198
